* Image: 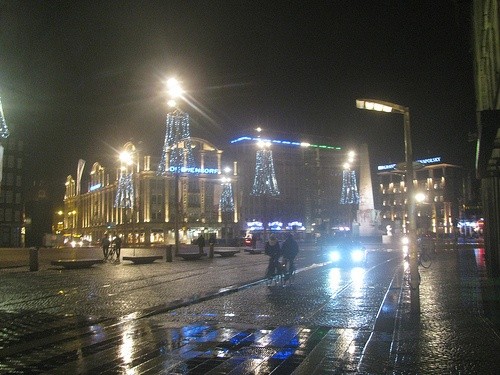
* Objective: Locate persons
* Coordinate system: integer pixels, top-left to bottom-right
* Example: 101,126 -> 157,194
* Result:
100,234 -> 110,258
454,226 -> 459,240
209,234 -> 216,246
113,235 -> 122,258
280,235 -> 298,274
195,235 -> 205,254
264,233 -> 283,278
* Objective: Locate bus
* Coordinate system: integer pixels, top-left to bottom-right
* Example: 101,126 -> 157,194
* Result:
244,229 -> 307,254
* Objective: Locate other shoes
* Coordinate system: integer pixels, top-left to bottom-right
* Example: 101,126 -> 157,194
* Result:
289,273 -> 292,276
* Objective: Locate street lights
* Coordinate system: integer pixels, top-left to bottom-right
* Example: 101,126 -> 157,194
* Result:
220,166 -> 231,246
119,150 -> 136,248
256,141 -> 272,243
165,76 -> 183,259
356,97 -> 421,315
343,150 -> 357,240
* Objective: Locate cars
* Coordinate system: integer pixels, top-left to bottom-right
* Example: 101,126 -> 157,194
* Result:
329,241 -> 368,266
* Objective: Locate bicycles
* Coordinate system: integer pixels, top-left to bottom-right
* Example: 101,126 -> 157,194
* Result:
264,256 -> 296,288
105,243 -> 116,260
